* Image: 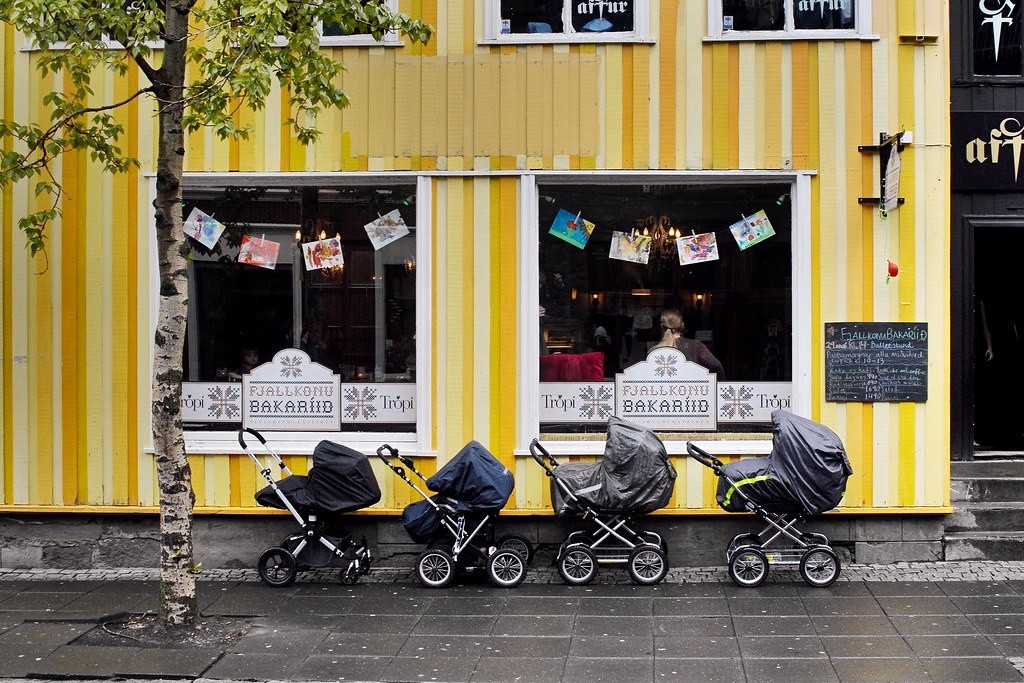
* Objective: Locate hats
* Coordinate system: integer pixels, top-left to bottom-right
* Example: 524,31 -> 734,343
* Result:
594,326 -> 609,337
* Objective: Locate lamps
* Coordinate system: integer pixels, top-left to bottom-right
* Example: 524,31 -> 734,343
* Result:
632,289 -> 651,296
294,218 -> 344,280
695,292 -> 705,305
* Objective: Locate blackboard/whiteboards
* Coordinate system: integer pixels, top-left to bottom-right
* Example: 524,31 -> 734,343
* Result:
824,322 -> 929,403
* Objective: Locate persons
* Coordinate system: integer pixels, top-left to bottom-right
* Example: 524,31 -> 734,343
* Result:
274,317 -> 342,369
240,344 -> 264,375
647,308 -> 724,381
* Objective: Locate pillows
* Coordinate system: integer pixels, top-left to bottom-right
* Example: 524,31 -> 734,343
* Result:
541,351 -> 604,383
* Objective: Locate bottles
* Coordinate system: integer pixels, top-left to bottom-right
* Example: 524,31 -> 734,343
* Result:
746,553 -> 781,562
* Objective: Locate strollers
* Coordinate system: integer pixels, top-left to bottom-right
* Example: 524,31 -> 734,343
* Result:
685,408 -> 852,589
531,415 -> 678,587
238,426 -> 381,589
377,439 -> 531,590
